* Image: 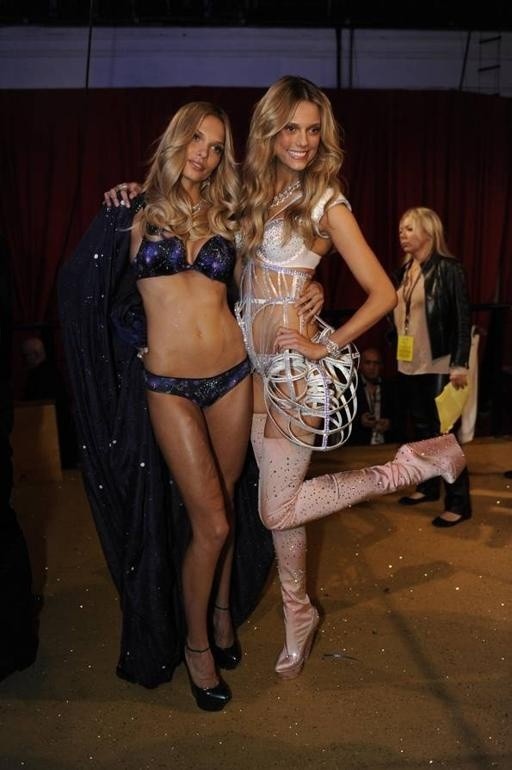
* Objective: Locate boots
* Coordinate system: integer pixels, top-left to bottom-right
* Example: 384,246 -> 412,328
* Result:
251,411 -> 321,680
257,428 -> 467,532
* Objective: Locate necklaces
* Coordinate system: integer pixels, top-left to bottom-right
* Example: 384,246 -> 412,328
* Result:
270,179 -> 302,209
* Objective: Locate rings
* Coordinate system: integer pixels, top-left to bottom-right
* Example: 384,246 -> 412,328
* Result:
117,185 -> 130,191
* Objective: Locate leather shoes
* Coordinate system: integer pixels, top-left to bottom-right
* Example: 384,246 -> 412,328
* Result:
398,491 -> 441,506
431,508 -> 472,528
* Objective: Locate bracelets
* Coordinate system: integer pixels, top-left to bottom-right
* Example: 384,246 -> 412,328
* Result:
322,336 -> 339,359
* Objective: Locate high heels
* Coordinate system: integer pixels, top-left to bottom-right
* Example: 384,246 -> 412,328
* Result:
210,602 -> 242,670
179,638 -> 232,712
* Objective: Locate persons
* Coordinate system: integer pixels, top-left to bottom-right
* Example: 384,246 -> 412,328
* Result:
56,93 -> 258,713
96,74 -> 469,681
342,341 -> 407,448
381,205 -> 481,527
